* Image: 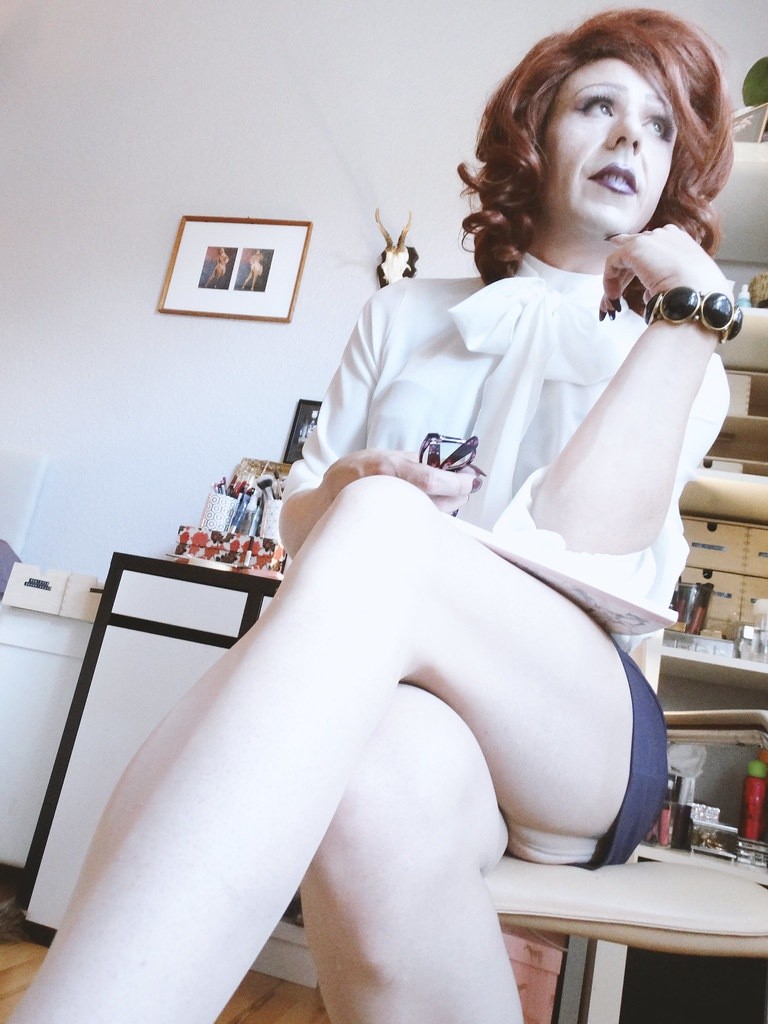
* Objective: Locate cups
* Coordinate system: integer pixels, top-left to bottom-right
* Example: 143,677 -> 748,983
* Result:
260,499 -> 284,550
200,493 -> 239,532
677,583 -> 714,635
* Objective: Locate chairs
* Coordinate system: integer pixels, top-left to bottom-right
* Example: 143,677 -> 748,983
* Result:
487,639 -> 768,1024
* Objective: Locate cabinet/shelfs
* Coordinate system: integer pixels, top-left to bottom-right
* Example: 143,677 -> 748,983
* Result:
15,551 -> 282,947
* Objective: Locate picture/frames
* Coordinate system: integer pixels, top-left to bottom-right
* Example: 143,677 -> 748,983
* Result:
157,215 -> 314,323
283,399 -> 322,464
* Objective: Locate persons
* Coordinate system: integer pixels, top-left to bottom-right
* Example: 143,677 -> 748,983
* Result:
241,249 -> 265,292
9,8 -> 745,1024
204,245 -> 230,289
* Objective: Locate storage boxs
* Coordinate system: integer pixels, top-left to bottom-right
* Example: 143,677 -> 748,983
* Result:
665,515 -> 768,634
174,525 -> 286,572
500,924 -> 566,1024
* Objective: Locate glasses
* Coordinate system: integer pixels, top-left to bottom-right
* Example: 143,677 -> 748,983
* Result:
418,432 -> 487,518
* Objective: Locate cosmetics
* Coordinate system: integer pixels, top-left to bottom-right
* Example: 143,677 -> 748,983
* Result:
669,582 -> 715,634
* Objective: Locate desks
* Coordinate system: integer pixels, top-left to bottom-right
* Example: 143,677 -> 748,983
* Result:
579,647 -> 768,1024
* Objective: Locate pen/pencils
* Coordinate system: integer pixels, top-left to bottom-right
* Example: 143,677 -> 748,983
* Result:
213,476 -> 256,501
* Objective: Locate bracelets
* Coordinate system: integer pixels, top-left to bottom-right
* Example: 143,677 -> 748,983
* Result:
645,285 -> 743,344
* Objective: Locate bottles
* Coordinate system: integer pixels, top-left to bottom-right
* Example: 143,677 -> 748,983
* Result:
671,805 -> 693,850
737,759 -> 767,840
753,598 -> 768,664
737,284 -> 752,309
